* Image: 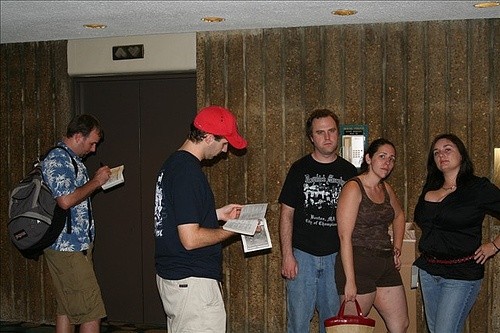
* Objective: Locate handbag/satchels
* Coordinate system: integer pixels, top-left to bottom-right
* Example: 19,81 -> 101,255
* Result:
325,298 -> 376,333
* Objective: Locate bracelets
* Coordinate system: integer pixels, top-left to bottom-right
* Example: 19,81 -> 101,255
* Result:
491,242 -> 500,250
394,246 -> 401,256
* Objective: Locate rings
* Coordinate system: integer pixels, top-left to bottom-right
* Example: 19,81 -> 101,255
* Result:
482,254 -> 485,257
284,274 -> 288,276
481,251 -> 483,253
352,297 -> 356,298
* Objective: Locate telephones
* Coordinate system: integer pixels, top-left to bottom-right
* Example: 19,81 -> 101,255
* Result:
341,134 -> 366,169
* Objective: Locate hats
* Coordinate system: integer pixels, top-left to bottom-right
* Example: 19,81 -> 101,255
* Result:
192,104 -> 249,150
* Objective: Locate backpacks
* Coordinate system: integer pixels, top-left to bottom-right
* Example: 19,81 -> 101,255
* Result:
7,146 -> 78,262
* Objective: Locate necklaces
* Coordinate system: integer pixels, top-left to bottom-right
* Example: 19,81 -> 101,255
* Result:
441,186 -> 457,190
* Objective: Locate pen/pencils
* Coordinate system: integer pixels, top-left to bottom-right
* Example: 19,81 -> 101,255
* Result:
100,162 -> 103,170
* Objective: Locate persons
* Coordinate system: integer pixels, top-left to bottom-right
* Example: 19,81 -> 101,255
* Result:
41,114 -> 114,333
277,108 -> 358,333
334,139 -> 410,333
412,133 -> 500,333
152,105 -> 263,333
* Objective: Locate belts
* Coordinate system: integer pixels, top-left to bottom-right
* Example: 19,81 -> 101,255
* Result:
426,254 -> 476,264
